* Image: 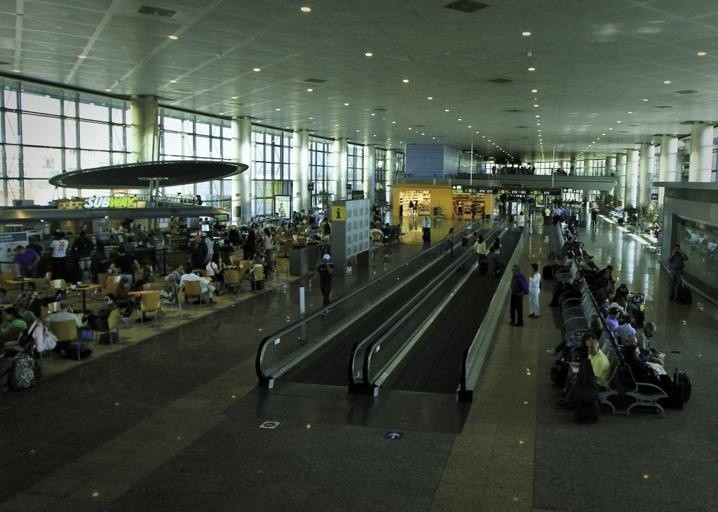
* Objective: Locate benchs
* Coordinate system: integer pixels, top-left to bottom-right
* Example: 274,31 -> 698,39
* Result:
548,216 -> 668,417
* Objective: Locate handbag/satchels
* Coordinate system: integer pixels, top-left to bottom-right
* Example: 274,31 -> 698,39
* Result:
680,373 -> 691,402
14,355 -> 34,389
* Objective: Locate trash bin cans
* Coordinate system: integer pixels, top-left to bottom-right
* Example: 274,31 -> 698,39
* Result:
623,211 -> 628,222
289,244 -> 308,276
309,242 -> 320,270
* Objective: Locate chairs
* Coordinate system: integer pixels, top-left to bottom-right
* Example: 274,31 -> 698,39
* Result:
255,209 -> 330,257
0,248 -> 290,361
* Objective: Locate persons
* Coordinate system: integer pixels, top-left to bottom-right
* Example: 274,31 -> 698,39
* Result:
541,201 -> 664,240
370,205 -> 402,243
508,236 -> 687,409
409,201 -> 526,273
0,205 -> 336,357
492,162 -> 567,175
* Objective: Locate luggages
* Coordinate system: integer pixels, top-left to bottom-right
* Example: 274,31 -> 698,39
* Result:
679,273 -> 692,306
670,351 -> 685,409
574,364 -> 599,423
